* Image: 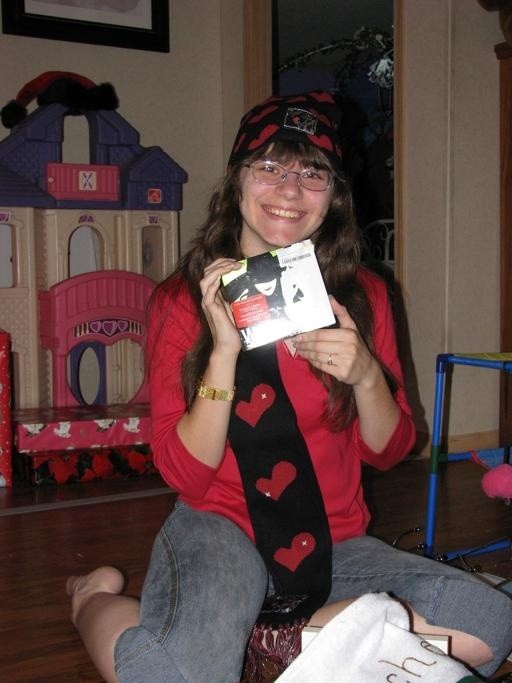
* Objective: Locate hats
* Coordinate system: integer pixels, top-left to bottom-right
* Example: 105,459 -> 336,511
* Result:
224,87 -> 348,171
243,251 -> 288,282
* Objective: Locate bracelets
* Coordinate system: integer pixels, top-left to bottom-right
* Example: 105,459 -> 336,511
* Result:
194,379 -> 237,401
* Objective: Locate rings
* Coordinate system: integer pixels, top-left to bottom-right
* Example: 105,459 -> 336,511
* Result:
327,352 -> 335,365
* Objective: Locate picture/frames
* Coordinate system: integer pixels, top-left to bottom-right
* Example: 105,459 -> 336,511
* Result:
0,0 -> 170,53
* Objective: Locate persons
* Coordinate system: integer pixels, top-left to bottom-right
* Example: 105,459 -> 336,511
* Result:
240,251 -> 304,325
64,95 -> 510,682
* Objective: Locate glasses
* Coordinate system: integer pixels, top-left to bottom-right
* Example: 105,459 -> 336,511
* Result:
240,157 -> 339,194
